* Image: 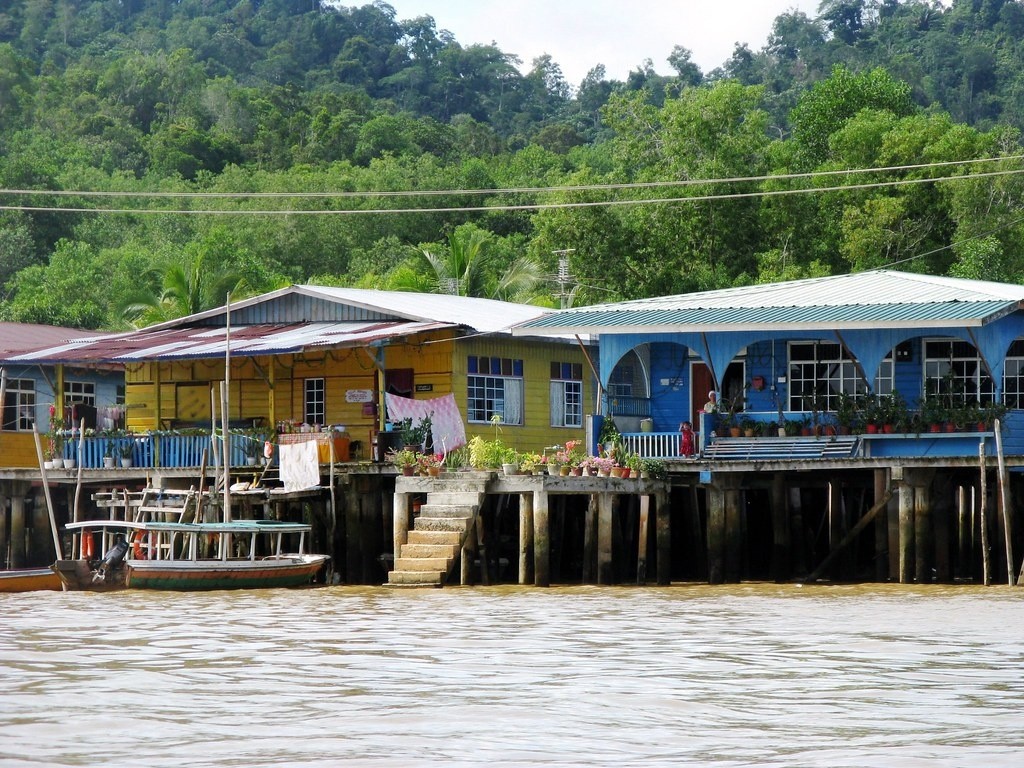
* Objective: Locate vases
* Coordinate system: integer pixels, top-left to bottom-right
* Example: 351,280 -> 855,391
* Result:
572,467 -> 583,476
560,466 -> 570,475
612,466 -> 622,477
586,465 -> 599,475
547,463 -> 558,475
419,470 -> 428,476
599,469 -> 610,476
427,467 -> 438,476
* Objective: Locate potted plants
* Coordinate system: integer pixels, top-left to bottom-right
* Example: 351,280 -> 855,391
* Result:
43,418 -> 75,469
103,441 -> 116,466
502,448 -> 545,475
118,442 -> 135,467
710,369 -> 1017,437
619,442 -> 665,478
445,435 -> 487,472
384,411 -> 434,476
233,432 -> 276,467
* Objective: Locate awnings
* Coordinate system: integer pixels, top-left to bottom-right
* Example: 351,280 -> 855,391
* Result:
1,322 -> 462,460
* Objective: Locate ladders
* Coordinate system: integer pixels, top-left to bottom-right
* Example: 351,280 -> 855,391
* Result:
124,482 -> 197,560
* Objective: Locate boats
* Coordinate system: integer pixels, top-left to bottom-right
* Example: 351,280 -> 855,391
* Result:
49,518 -> 330,593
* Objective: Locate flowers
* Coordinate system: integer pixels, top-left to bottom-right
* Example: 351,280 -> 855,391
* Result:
415,452 -> 442,471
539,440 -> 619,474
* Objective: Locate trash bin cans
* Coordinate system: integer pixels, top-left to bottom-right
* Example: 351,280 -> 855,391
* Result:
640,419 -> 653,432
377,430 -> 405,463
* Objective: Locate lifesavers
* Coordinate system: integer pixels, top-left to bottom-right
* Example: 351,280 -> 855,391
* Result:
133,528 -> 156,559
83,530 -> 93,559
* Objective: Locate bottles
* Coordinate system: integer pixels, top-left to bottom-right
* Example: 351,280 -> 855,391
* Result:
277,419 -> 301,433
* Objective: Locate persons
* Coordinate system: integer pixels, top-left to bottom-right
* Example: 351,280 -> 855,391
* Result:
704,390 -> 725,412
678,420 -> 696,459
